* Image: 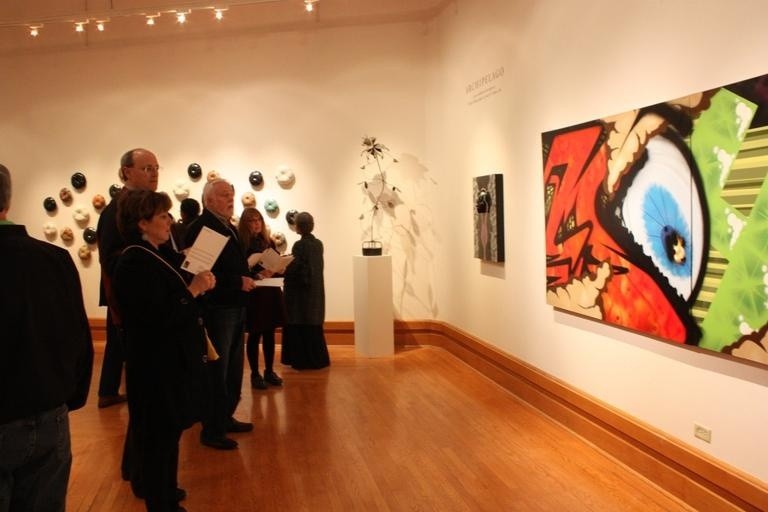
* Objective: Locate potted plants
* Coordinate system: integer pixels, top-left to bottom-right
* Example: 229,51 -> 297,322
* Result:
355,133 -> 398,256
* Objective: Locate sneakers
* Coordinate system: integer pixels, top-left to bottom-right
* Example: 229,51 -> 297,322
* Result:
252,377 -> 266,389
229,418 -> 254,432
201,437 -> 238,449
265,371 -> 282,384
136,487 -> 186,501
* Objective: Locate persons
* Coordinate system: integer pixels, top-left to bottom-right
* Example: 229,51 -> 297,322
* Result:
183,178 -> 255,451
171,198 -> 201,253
0,164 -> 94,512
112,188 -> 217,512
280,212 -> 330,371
237,206 -> 287,390
95,148 -> 160,410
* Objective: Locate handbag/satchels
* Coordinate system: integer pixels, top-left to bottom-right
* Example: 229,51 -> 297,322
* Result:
204,327 -> 220,361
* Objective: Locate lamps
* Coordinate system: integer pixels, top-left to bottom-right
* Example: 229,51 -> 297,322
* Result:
19,0 -> 230,38
304,0 -> 317,10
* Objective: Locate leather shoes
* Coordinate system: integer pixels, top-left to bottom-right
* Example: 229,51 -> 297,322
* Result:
98,393 -> 127,408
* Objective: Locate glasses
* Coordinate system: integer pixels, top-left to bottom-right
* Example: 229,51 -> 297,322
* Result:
128,166 -> 158,177
